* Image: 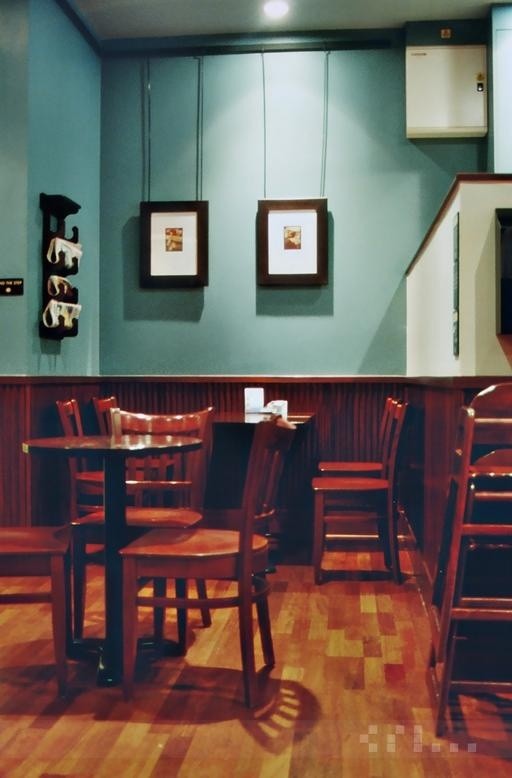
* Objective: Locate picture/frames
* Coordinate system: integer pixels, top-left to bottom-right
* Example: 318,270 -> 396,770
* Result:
257,200 -> 328,286
140,201 -> 208,288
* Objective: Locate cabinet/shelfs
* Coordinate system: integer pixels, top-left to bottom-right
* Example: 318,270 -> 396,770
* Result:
40,193 -> 81,341
405,46 -> 488,139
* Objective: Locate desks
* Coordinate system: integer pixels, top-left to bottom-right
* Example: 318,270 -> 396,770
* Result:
21,435 -> 203,688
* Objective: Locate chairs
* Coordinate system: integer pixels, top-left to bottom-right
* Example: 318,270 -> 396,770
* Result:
312,397 -> 408,585
0,526 -> 75,694
56,395 -> 284,706
